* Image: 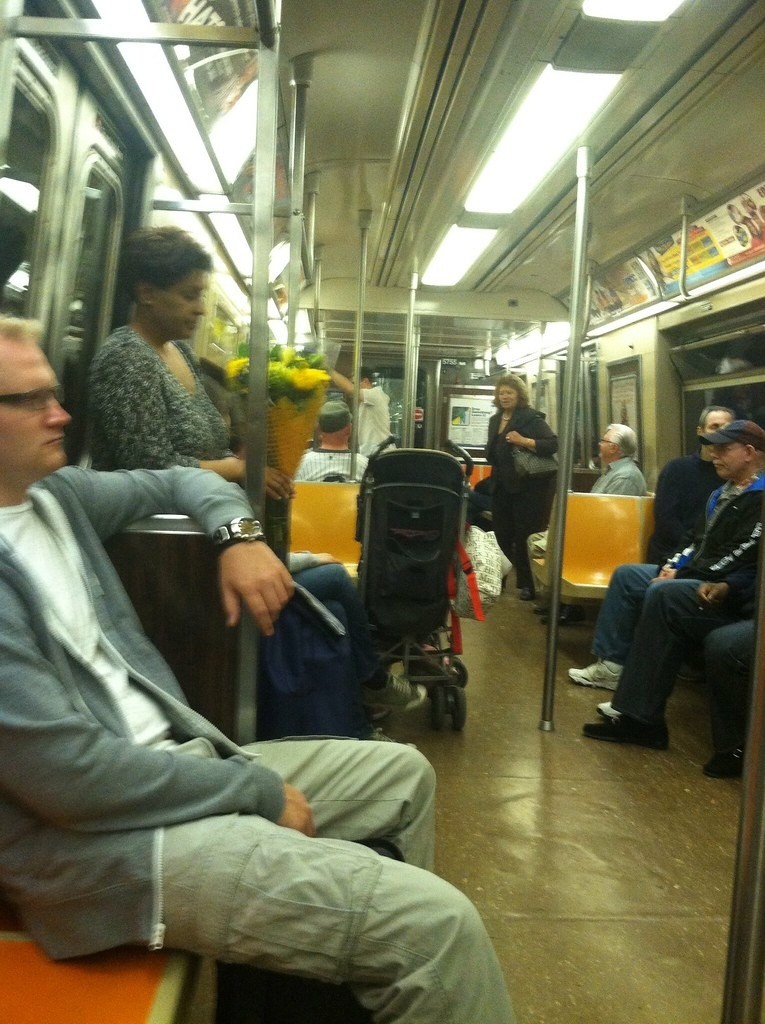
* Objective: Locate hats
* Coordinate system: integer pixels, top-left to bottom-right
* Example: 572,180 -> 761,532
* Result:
319,401 -> 352,433
698,420 -> 765,451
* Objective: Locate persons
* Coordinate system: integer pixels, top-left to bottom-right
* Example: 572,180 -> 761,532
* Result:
484,375 -> 558,600
647,405 -> 737,681
568,419 -> 765,721
191,365 -> 427,753
582,559 -> 758,776
0,319 -> 516,1024
526,423 -> 646,624
86,226 -> 296,501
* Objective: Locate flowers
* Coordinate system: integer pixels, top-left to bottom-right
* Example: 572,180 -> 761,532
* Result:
226,343 -> 333,574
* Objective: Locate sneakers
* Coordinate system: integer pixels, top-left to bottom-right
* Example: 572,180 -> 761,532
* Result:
596,701 -> 621,717
365,728 -> 418,752
568,658 -> 624,690
360,674 -> 428,711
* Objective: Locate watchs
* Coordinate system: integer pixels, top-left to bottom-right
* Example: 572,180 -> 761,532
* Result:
214,515 -> 264,546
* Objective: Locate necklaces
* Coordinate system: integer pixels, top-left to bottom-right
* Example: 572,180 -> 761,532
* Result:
502,412 -> 510,421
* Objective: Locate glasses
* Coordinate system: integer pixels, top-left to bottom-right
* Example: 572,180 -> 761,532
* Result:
600,438 -> 620,447
1,384 -> 66,410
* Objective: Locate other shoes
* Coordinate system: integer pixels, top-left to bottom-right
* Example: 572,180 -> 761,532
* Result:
534,606 -> 550,615
541,613 -> 586,625
583,714 -> 669,750
703,747 -> 742,777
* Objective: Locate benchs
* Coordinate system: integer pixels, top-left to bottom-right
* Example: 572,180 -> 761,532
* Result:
0,464 -> 658,1024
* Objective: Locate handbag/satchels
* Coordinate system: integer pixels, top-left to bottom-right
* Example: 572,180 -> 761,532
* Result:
511,446 -> 557,481
455,520 -> 503,622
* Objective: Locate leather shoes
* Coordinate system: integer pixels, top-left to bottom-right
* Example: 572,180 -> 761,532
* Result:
519,587 -> 535,601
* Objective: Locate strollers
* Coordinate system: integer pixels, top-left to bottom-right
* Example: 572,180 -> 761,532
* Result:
352,434 -> 472,734
